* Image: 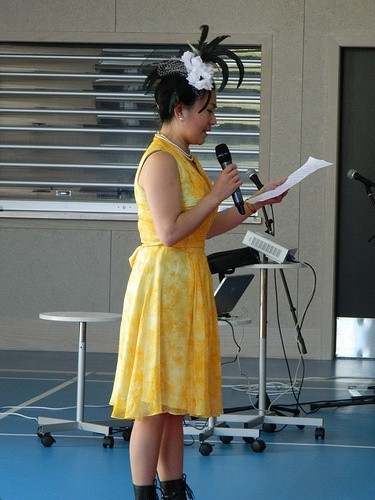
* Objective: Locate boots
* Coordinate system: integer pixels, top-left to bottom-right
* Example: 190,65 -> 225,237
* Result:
131,478 -> 166,500
158,474 -> 195,500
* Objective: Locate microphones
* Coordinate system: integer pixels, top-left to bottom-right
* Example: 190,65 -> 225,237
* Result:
347,167 -> 375,187
247,169 -> 264,190
216,144 -> 245,216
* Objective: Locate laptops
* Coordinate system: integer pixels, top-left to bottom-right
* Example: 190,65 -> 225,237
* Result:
214,274 -> 254,315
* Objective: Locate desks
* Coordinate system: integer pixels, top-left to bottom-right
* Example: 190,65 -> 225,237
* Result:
214,261 -> 326,443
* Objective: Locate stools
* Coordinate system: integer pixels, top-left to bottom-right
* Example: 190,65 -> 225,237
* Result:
36,310 -> 132,448
182,317 -> 266,456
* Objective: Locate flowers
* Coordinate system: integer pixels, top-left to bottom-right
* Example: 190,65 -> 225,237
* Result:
182,50 -> 215,90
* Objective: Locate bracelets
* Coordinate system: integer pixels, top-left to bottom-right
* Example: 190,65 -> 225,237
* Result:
246,198 -> 257,213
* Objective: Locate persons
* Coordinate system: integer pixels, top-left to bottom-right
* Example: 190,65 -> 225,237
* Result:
110,63 -> 286,500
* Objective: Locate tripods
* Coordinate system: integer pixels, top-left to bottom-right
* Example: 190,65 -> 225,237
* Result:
222,206 -> 307,417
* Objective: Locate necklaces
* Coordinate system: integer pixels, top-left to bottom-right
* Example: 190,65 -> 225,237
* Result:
154,132 -> 193,162
